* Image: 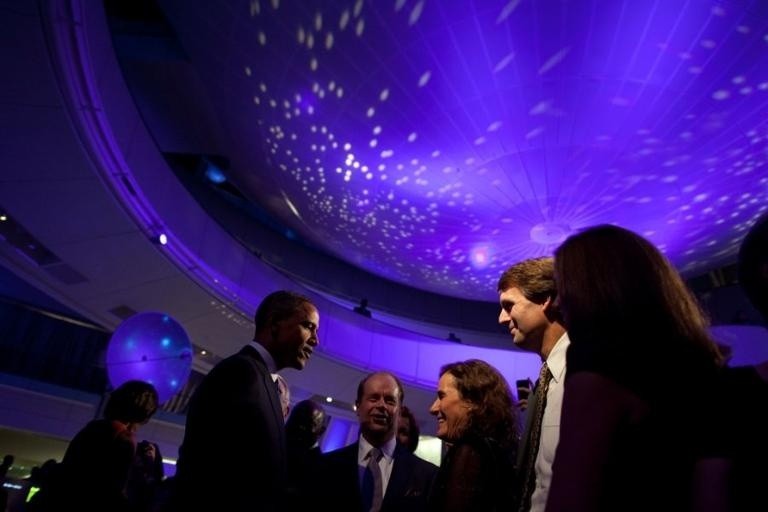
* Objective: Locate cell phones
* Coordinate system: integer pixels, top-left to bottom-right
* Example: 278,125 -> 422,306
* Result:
516,380 -> 530,400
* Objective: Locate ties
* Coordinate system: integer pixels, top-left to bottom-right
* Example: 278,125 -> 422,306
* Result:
512,361 -> 555,511
361,445 -> 384,512
